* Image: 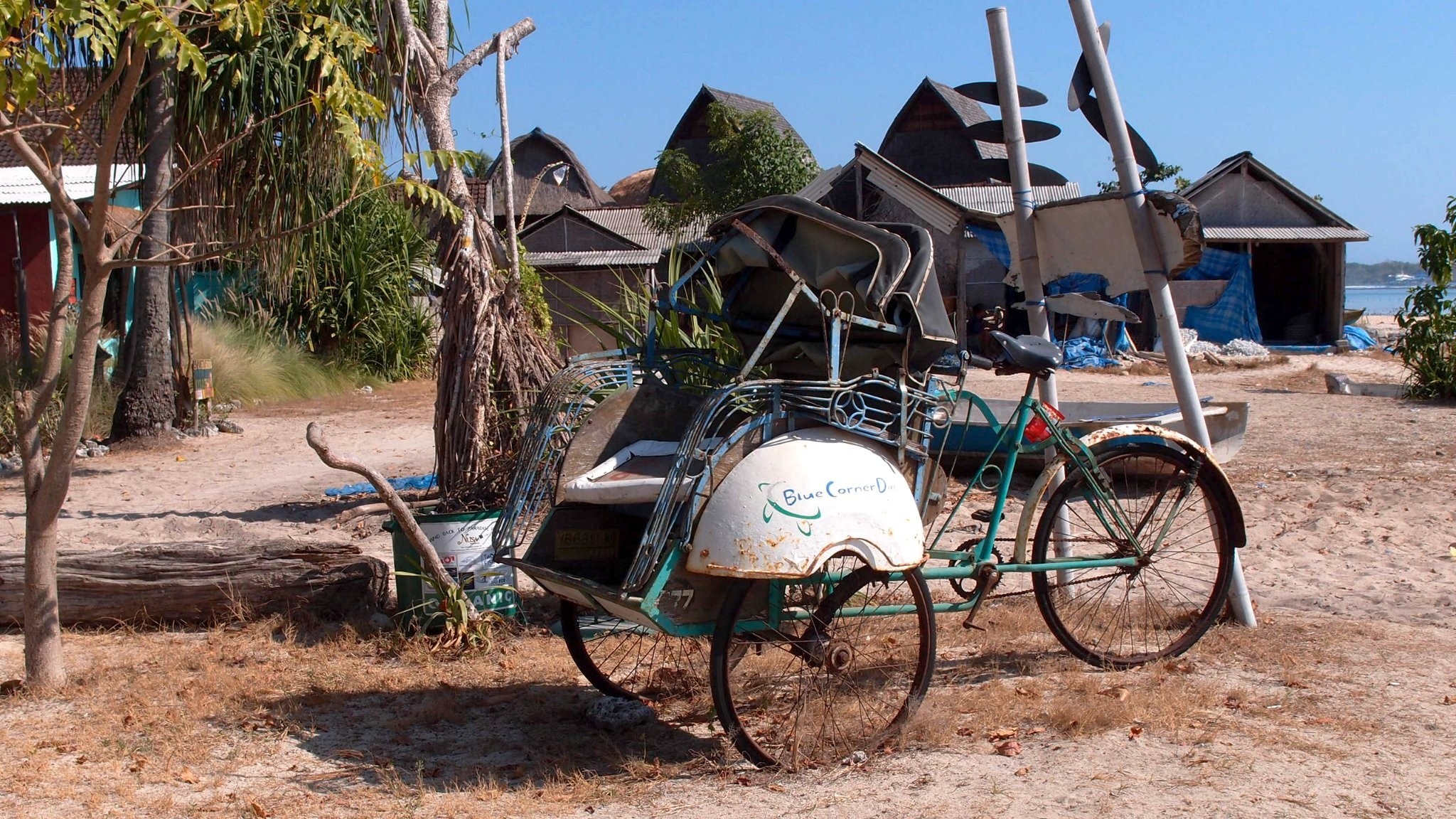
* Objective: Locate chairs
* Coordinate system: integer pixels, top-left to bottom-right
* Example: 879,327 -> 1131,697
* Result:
558,379 -> 775,510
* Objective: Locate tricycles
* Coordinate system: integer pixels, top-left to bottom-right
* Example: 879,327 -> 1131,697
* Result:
501,191 -> 1252,775
